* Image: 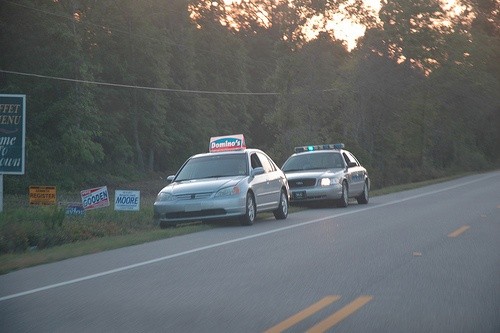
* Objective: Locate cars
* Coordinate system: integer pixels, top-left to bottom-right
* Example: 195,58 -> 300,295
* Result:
280,143 -> 372,209
153,133 -> 290,231
